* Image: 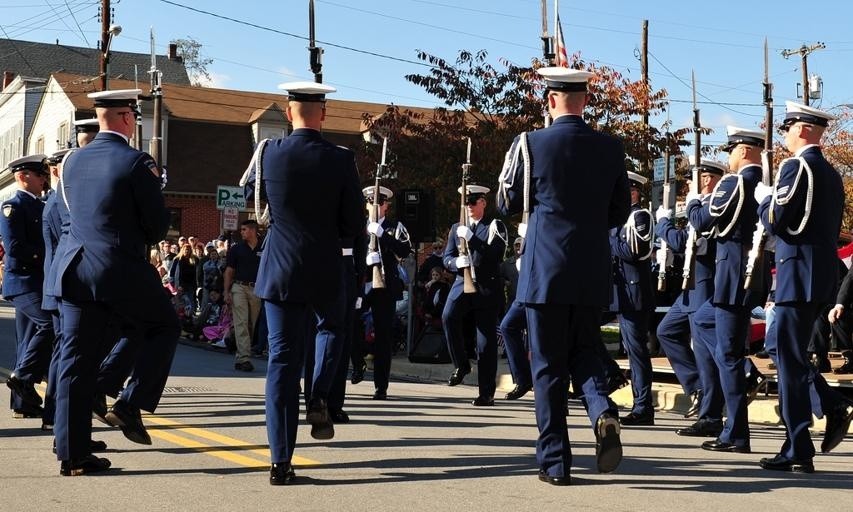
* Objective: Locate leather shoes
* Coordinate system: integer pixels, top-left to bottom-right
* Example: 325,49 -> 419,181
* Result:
604,366 -> 629,395
5,371 -> 151,476
269,357 -> 389,485
625,348 -> 853,485
448,366 -> 471,386
471,395 -> 495,407
234,360 -> 254,372
538,469 -> 572,486
504,383 -> 531,399
593,409 -> 622,474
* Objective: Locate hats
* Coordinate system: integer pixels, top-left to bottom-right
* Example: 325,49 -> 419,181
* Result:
457,185 -> 490,203
277,80 -> 336,103
536,67 -> 594,99
7,88 -> 142,174
362,185 -> 394,201
626,98 -> 835,190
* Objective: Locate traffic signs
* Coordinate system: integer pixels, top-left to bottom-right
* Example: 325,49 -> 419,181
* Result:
214,183 -> 248,211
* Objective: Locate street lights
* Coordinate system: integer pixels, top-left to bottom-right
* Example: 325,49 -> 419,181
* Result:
97,24 -> 124,91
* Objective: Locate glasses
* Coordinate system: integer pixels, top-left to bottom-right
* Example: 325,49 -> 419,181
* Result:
369,198 -> 384,206
469,198 -> 477,206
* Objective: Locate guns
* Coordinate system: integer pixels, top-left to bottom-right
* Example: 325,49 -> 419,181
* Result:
540,0 -> 556,128
657,145 -> 670,291
763,36 -> 775,186
368,137 -> 388,289
692,68 -> 702,194
460,137 -> 476,293
148,27 -> 164,182
307,0 -> 322,84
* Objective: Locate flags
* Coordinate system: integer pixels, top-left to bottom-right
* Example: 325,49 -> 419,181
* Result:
540,15 -> 567,117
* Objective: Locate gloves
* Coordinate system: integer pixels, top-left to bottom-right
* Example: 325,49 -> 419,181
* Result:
517,223 -> 528,239
456,225 -> 474,243
655,248 -> 674,266
752,183 -> 774,206
684,192 -> 702,207
365,252 -> 380,266
655,205 -> 673,224
367,222 -> 384,237
455,256 -> 469,269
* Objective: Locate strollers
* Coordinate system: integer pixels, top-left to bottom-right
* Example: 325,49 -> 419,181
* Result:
396,254 -> 454,359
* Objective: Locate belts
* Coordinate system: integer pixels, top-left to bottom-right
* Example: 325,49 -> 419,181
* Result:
234,281 -> 254,287
